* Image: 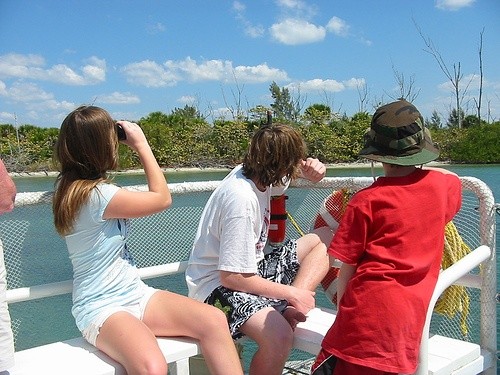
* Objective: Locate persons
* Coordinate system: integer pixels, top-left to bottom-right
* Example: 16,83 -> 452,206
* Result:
311,101 -> 462,375
185,124 -> 335,375
53,105 -> 244,375
0,159 -> 16,375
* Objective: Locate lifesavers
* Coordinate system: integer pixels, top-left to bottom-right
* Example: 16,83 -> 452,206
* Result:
312,188 -> 355,307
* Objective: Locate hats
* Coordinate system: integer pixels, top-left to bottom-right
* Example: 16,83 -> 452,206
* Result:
357,101 -> 440,166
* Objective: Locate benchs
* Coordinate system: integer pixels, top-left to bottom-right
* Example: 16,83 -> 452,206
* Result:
0,175 -> 498,375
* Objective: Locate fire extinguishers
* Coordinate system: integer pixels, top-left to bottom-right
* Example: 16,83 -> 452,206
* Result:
268,193 -> 289,243
